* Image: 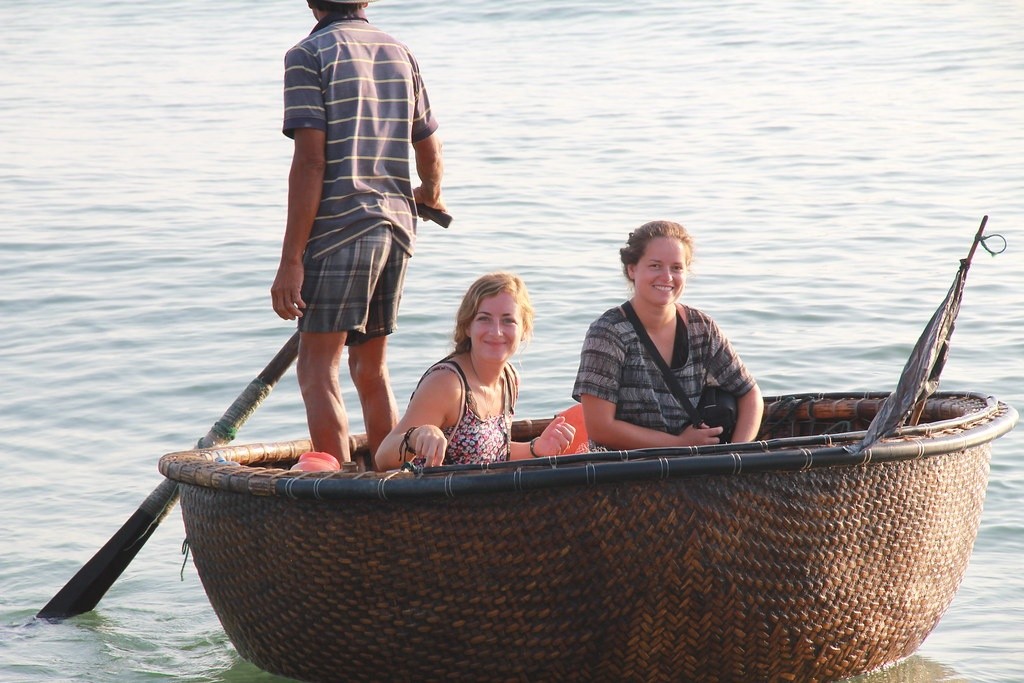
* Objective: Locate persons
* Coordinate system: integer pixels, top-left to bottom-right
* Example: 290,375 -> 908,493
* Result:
374,274 -> 576,472
270,0 -> 447,469
572,221 -> 765,451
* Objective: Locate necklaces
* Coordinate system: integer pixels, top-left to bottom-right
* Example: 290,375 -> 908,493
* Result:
470,352 -> 498,418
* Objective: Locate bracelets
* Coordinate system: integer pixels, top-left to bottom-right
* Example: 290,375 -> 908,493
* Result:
398,427 -> 418,463
530,437 -> 540,458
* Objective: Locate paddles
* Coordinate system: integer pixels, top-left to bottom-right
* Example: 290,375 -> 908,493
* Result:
31,328 -> 304,621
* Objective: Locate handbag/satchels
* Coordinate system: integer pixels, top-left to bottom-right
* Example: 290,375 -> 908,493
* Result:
692,384 -> 739,444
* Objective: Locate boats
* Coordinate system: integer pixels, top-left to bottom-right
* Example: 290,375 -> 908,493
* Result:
157,221 -> 1020,682
